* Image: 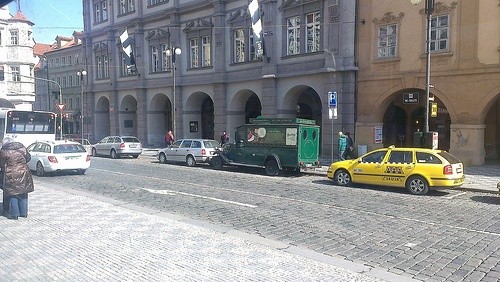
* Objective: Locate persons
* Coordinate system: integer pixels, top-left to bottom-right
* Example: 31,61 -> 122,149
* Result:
164,130 -> 173,146
343,132 -> 357,159
0,137 -> 34,220
337,131 -> 346,161
221,131 -> 229,144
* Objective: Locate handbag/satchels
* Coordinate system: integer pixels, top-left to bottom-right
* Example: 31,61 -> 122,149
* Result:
349,146 -> 355,152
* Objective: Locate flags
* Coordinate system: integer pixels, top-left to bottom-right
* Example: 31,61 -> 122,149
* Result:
248,0 -> 265,58
120,29 -> 136,69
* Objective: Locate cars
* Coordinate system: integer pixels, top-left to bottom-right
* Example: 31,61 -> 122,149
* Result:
91,135 -> 143,159
63,138 -> 93,156
327,144 -> 465,195
156,138 -> 223,167
25,140 -> 91,177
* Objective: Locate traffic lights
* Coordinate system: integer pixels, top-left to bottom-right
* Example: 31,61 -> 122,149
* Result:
0,66 -> 4,81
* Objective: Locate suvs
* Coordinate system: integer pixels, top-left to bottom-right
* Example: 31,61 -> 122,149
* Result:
210,115 -> 320,176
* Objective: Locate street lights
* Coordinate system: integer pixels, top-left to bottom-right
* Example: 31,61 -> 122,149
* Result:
76,69 -> 87,146
165,45 -> 182,143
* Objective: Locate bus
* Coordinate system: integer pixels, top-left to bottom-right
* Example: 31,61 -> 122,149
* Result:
0,107 -> 56,153
